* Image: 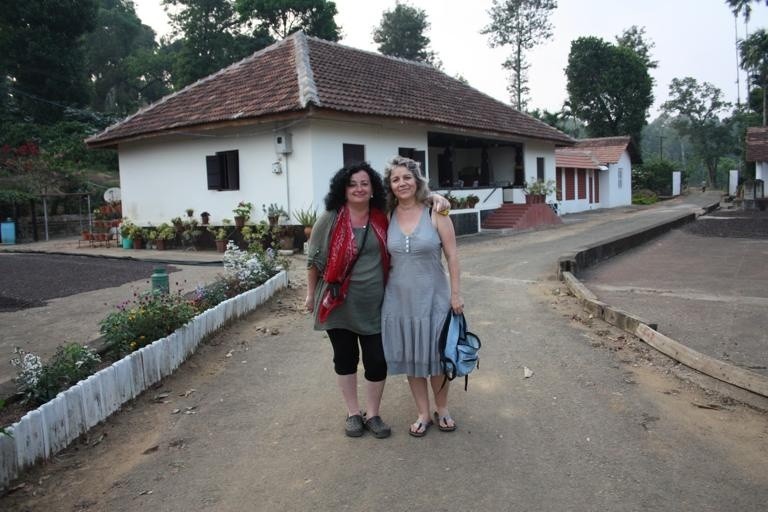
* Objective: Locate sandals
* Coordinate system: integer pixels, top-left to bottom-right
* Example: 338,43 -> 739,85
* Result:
346,411 -> 391,438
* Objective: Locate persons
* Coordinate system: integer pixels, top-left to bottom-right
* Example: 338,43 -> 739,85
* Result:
381,157 -> 464,438
702,179 -> 707,192
304,161 -> 451,439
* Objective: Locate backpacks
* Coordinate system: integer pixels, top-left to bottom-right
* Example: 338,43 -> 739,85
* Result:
440,308 -> 479,381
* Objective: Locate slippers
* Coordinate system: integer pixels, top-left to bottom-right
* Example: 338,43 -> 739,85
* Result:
408,411 -> 456,436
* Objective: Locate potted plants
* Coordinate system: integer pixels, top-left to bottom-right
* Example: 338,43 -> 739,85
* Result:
523,176 -> 560,204
444,191 -> 480,210
80,199 -> 318,250
